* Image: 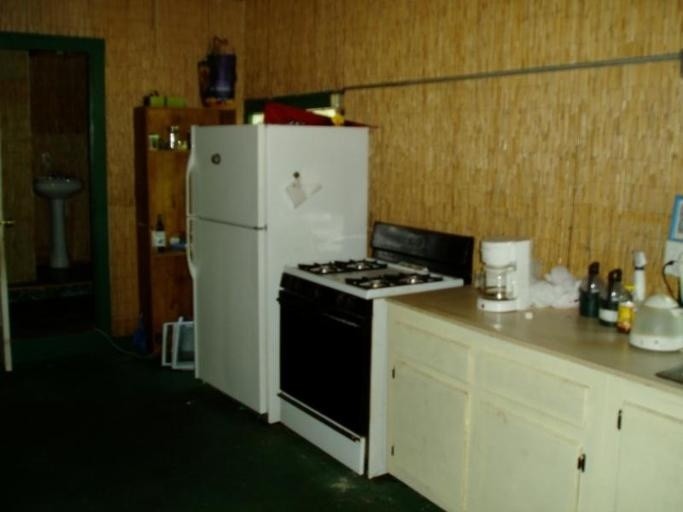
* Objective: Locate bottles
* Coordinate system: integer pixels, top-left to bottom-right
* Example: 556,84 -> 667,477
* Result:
616,285 -> 636,333
156,214 -> 165,252
170,126 -> 179,150
598,268 -> 632,327
580,262 -> 604,318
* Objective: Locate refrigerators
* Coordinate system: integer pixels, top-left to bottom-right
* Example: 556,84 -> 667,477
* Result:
186,122 -> 369,417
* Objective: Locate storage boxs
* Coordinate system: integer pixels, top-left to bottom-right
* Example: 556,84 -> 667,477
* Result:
598,320 -> 682,511
388,284 -> 595,512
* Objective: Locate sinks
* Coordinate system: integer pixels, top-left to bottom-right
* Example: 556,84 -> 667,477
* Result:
35,177 -> 83,199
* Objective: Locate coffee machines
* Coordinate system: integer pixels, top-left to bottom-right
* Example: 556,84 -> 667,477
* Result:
475,238 -> 531,312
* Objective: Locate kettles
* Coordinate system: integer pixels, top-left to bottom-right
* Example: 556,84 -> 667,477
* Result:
630,291 -> 681,352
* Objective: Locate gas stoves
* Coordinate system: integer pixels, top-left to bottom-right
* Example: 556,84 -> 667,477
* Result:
280,219 -> 476,326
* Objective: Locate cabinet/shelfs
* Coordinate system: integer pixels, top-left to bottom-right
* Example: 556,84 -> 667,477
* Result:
130,106 -> 237,354
